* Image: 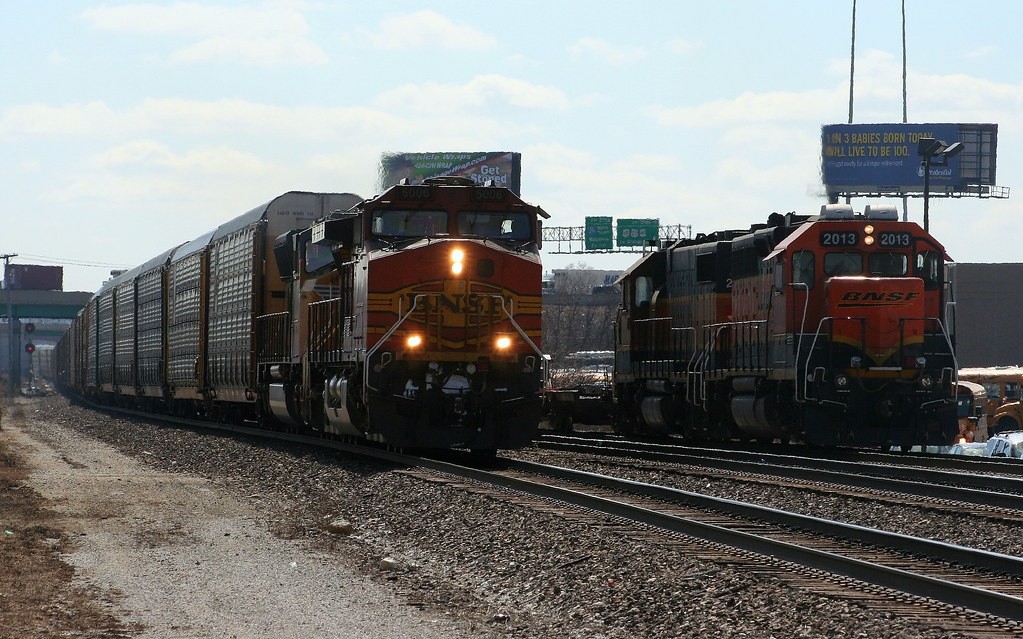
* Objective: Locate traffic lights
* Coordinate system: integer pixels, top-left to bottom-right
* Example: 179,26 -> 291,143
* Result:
25,343 -> 35,354
25,323 -> 35,335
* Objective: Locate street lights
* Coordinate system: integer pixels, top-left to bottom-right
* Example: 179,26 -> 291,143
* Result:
918,136 -> 962,235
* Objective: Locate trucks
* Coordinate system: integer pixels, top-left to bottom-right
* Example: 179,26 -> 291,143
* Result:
954,365 -> 1023,445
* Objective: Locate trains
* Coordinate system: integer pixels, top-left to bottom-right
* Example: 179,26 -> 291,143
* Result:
50,176 -> 551,461
612,204 -> 960,458
